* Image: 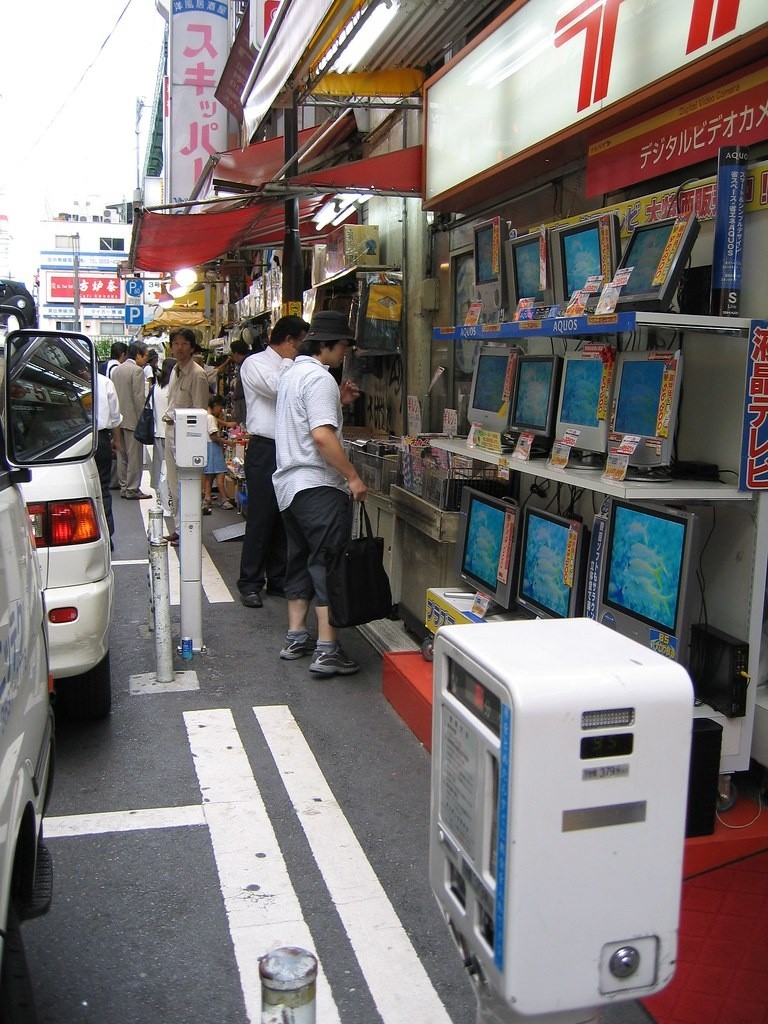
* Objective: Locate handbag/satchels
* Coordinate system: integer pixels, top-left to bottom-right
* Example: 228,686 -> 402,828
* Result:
134,384 -> 155,445
324,493 -> 392,628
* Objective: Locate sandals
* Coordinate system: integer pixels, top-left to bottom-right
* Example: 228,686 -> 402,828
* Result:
220,501 -> 233,509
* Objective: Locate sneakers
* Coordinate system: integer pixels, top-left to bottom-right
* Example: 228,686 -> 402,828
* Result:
309,641 -> 361,675
280,635 -> 317,660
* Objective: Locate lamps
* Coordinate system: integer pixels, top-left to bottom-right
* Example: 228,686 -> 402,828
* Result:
311,187 -> 373,232
169,272 -> 187,299
174,265 -> 210,289
158,282 -> 175,310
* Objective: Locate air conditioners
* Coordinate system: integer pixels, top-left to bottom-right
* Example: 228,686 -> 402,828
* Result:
103,209 -> 120,223
77,213 -> 93,222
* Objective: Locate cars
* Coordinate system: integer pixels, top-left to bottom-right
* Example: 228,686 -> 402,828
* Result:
0,273 -> 117,966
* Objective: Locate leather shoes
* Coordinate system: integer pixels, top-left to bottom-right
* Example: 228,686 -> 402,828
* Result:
240,590 -> 263,608
266,589 -> 286,597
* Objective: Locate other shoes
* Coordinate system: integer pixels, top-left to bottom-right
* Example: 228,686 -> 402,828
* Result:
121,493 -> 152,499
171,539 -> 180,547
202,500 -> 214,509
163,532 -> 179,541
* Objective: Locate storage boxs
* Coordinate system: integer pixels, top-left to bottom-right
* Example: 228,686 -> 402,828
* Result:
221,267 -> 282,327
311,224 -> 379,286
223,475 -> 236,498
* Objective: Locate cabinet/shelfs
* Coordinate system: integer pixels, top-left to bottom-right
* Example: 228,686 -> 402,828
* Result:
424,312 -> 758,757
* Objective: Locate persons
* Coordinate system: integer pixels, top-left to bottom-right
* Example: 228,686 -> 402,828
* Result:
97,341 -> 178,551
194,340 -> 251,509
272,311 -> 367,675
237,315 -> 311,607
162,329 -> 208,547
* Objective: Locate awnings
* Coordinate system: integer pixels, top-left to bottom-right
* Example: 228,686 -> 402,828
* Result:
128,0 -> 502,273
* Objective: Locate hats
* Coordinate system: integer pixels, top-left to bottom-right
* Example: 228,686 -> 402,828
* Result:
146,348 -> 158,361
302,310 -> 356,347
195,345 -> 208,352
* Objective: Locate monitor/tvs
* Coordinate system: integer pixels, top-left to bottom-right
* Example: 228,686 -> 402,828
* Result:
442,212 -> 700,673
